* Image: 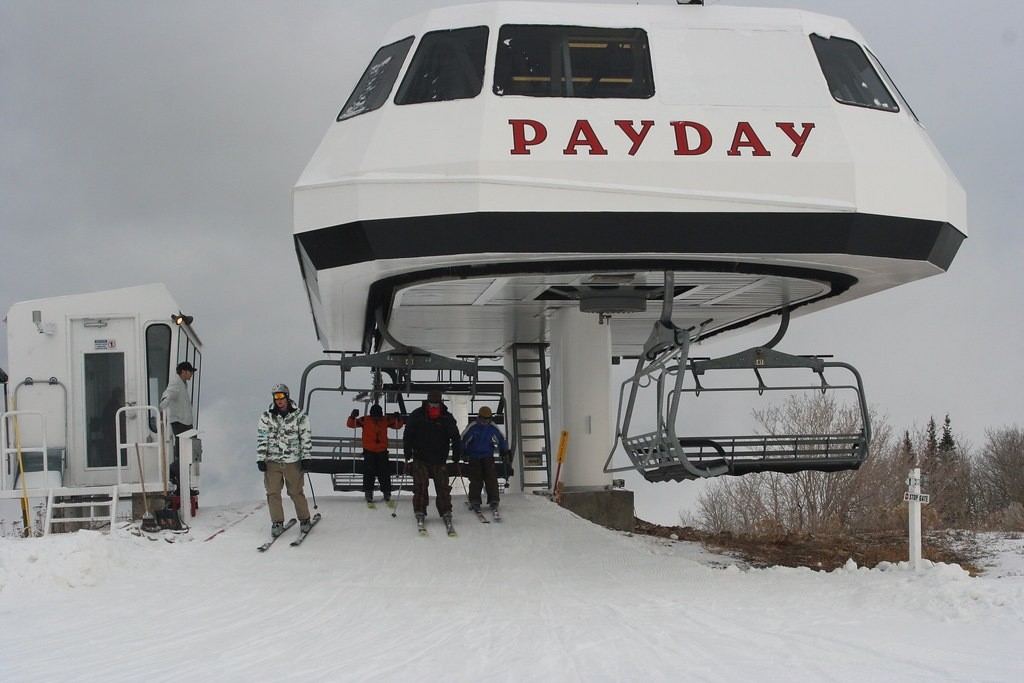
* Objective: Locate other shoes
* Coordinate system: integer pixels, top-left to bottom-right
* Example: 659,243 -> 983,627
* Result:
300,519 -> 310,529
272,522 -> 283,533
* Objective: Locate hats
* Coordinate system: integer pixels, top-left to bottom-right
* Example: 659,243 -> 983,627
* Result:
176,362 -> 197,371
370,404 -> 382,416
428,391 -> 441,403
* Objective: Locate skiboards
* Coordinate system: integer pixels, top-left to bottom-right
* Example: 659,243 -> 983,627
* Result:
417,517 -> 457,536
477,508 -> 502,523
367,500 -> 396,509
256,512 -> 321,550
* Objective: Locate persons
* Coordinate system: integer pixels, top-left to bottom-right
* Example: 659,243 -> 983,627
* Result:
403,390 -> 461,519
347,404 -> 403,502
101,387 -> 126,439
160,362 -> 200,496
461,407 -> 508,509
256,383 -> 312,536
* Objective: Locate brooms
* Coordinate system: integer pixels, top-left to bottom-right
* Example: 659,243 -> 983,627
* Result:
134,442 -> 161,533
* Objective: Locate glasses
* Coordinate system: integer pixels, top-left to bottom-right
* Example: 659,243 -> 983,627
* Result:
274,393 -> 285,400
429,402 -> 439,408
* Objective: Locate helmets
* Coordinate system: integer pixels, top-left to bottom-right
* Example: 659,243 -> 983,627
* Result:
272,384 -> 289,398
479,407 -> 491,418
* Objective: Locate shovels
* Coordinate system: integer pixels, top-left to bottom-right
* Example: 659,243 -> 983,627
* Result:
156,422 -> 181,530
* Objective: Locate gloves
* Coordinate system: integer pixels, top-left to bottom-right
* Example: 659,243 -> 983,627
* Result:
160,398 -> 169,409
302,459 -> 312,473
351,409 -> 359,419
404,448 -> 412,460
452,448 -> 460,461
394,412 -> 400,420
257,461 -> 267,471
